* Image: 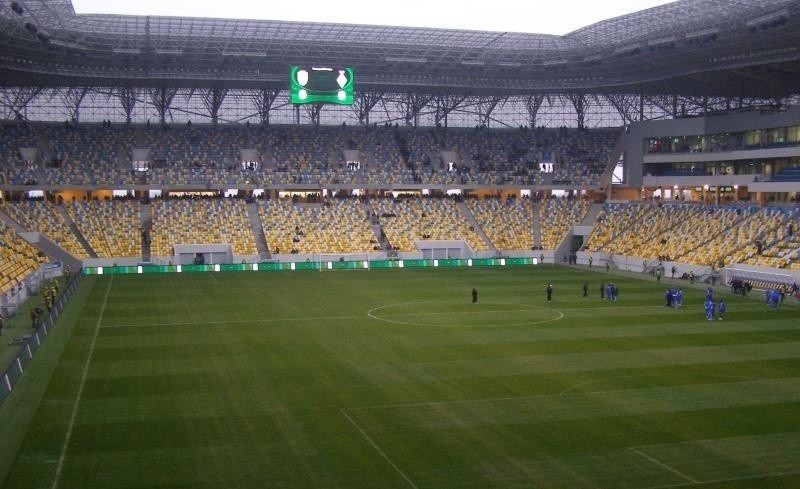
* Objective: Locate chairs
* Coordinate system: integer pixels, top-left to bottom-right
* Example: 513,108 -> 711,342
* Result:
2,200 -> 91,262
149,198 -> 258,258
539,197 -> 593,250
580,204 -> 800,271
463,197 -> 534,251
2,125 -> 417,186
257,199 -> 382,257
2,221 -> 52,296
396,126 -> 620,186
369,198 -> 491,252
62,199 -> 142,258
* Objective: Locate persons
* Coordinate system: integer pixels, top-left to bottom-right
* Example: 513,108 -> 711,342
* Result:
589,257 -> 593,267
563,240 -> 582,264
472,288 -> 478,304
601,283 -> 617,301
31,263 -> 71,328
728,277 -> 752,296
0,315 -> 4,336
765,287 -> 784,308
755,241 -> 762,254
643,259 -> 694,282
540,254 -> 544,264
665,287 -> 682,308
790,282 -> 800,304
606,260 -> 610,271
547,284 -> 553,302
583,282 -> 589,296
704,287 -> 725,321
